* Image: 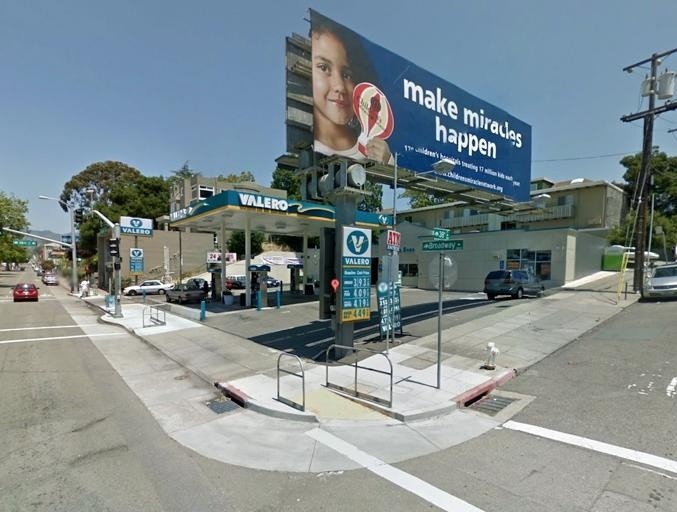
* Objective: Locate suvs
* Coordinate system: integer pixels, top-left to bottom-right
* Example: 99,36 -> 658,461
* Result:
188,278 -> 211,291
483,269 -> 544,299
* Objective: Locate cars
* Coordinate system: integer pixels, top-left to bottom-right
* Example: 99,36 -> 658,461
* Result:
643,265 -> 677,300
29,258 -> 52,276
122,279 -> 174,296
165,284 -> 206,304
622,246 -> 660,264
210,273 -> 280,289
41,274 -> 58,286
11,282 -> 40,302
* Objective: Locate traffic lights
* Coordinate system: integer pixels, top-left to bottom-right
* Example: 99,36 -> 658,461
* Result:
108,238 -> 117,257
72,209 -> 82,223
114,264 -> 120,270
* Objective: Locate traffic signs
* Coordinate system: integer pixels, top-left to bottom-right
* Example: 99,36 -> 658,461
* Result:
422,240 -> 463,252
12,239 -> 37,247
431,227 -> 451,240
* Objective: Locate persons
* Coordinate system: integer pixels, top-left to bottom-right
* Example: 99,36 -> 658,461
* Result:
310,8 -> 395,167
203,281 -> 209,304
81,279 -> 89,297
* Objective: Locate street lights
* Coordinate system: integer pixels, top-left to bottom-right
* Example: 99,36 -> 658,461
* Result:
391,150 -> 455,346
38,195 -> 80,296
